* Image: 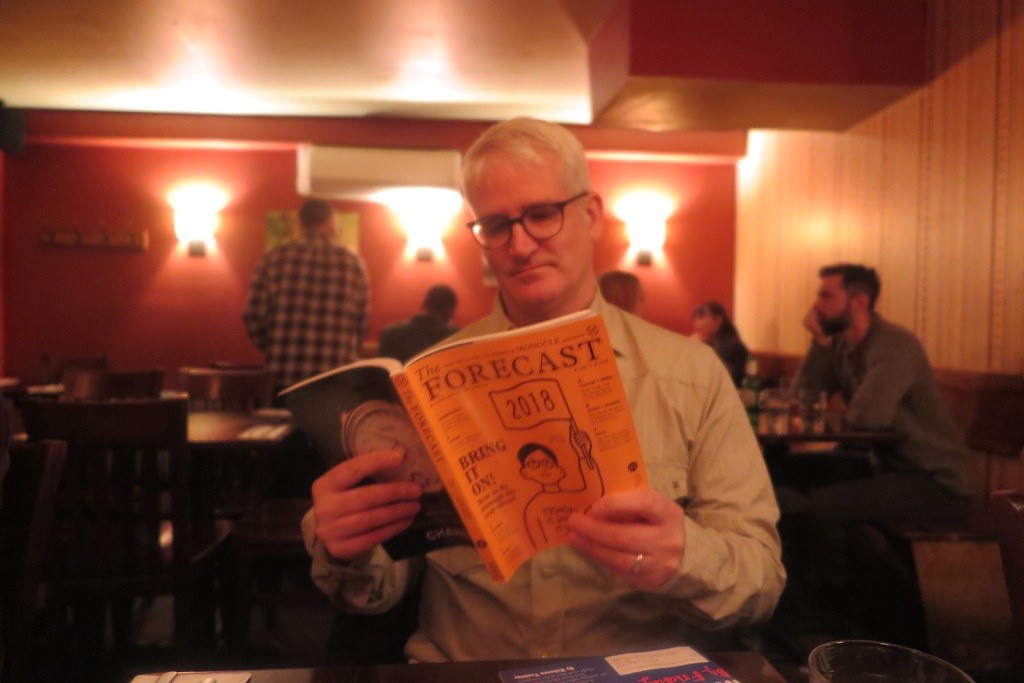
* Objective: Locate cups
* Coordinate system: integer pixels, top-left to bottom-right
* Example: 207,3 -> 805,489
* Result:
799,384 -> 827,433
808,640 -> 979,683
764,400 -> 791,435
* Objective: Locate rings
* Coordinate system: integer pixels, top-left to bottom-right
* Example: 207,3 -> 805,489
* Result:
632,554 -> 643,579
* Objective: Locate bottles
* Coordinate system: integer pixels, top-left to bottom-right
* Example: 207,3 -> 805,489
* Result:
742,356 -> 762,430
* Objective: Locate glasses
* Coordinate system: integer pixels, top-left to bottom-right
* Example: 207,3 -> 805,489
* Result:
467,191 -> 590,250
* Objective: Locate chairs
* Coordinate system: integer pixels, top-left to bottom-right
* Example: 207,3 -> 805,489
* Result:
0,361 -> 276,682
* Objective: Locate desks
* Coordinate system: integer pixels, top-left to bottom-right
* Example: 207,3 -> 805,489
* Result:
766,429 -> 909,628
0,405 -> 298,629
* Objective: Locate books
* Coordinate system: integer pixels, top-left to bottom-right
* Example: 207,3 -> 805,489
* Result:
279,309 -> 645,586
498,645 -> 742,683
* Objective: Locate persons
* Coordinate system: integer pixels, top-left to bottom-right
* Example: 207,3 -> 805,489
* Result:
303,118 -> 786,663
601,272 -> 640,311
378,285 -> 460,362
689,302 -> 747,388
778,264 -> 972,645
243,198 -> 368,406
